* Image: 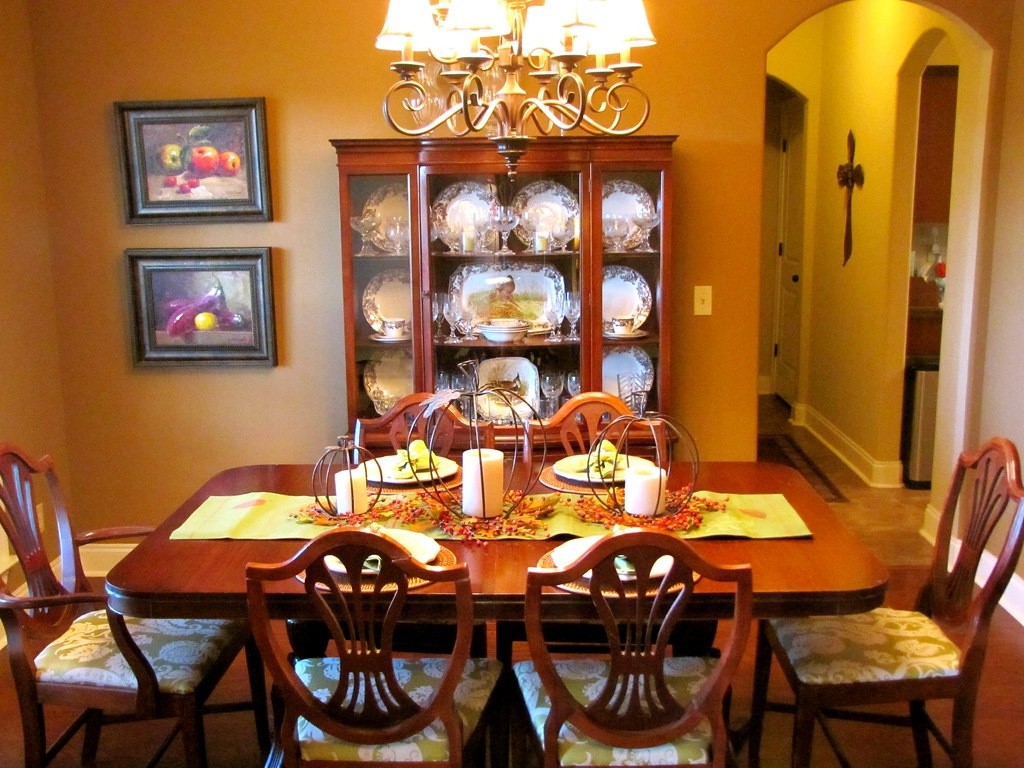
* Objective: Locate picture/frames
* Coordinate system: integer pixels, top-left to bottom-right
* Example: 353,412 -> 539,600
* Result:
113,97 -> 272,225
123,247 -> 277,369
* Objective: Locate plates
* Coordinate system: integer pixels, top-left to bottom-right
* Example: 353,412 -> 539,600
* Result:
361,268 -> 439,341
448,263 -> 567,334
552,536 -> 679,583
356,454 -> 458,484
595,343 -> 655,413
553,452 -> 656,483
596,266 -> 654,340
506,179 -> 584,249
429,180 -> 499,252
473,357 -> 541,421
322,528 -> 441,574
363,345 -> 415,417
360,183 -> 438,256
601,178 -> 655,249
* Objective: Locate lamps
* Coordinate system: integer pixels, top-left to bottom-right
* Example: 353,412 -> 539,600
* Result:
376,0 -> 656,182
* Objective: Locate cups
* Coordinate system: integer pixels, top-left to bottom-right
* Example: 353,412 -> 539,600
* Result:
611,317 -> 634,334
381,318 -> 404,337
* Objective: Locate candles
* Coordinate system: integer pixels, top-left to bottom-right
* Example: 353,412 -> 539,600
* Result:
461,447 -> 504,517
333,469 -> 367,515
624,464 -> 666,515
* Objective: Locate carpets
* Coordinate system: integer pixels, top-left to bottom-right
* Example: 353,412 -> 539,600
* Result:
758,433 -> 849,502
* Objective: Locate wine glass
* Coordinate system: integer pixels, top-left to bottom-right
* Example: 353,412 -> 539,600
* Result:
493,215 -> 520,255
432,370 -> 475,416
522,209 -> 556,252
538,370 -> 584,419
347,206 -> 661,256
350,213 -> 407,254
428,292 -> 478,343
603,213 -> 660,253
543,290 -> 581,343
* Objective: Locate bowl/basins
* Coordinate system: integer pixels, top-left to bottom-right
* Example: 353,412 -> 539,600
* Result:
476,318 -> 531,342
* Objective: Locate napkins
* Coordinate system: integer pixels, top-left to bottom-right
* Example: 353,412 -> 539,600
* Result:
577,439 -> 623,479
611,521 -> 644,573
365,522 -> 388,572
387,438 -> 439,477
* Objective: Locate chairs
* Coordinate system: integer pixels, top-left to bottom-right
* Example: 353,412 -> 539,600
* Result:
0,393 -> 1023,768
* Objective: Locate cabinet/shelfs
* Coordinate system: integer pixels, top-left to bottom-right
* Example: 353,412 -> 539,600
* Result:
330,136 -> 679,462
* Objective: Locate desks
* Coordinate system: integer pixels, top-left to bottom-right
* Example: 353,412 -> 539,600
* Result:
105,464 -> 889,668
